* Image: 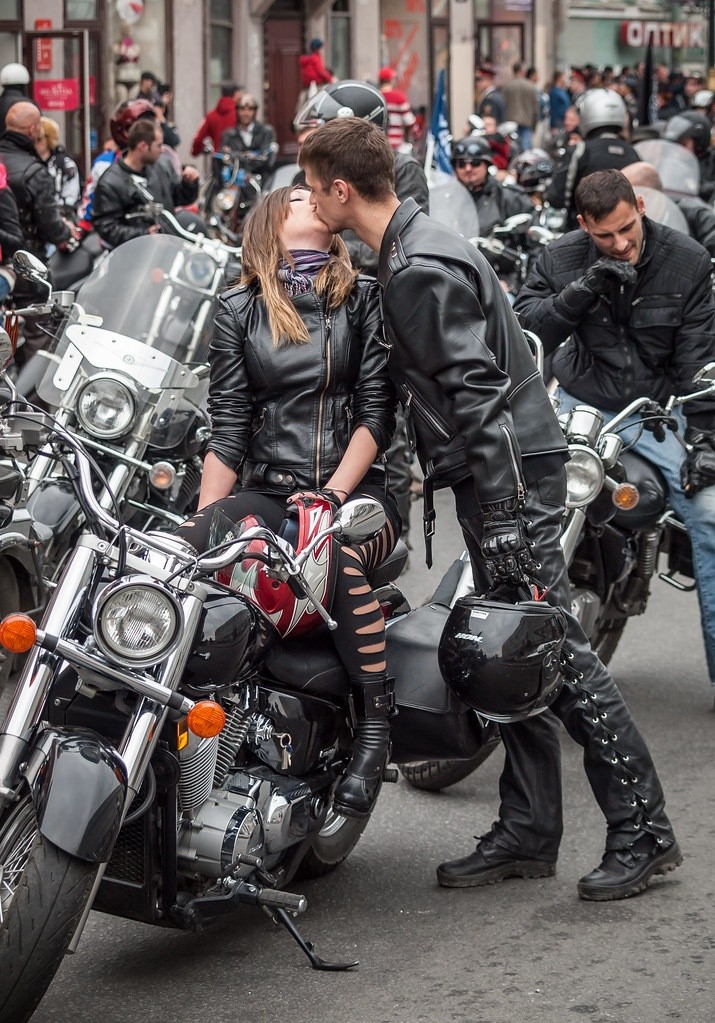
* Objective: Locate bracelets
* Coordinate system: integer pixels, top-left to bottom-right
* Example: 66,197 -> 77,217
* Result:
324,488 -> 348,496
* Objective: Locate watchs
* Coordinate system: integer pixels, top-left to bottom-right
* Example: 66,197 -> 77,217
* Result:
322,489 -> 341,507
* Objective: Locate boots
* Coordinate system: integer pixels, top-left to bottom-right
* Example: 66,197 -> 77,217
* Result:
332,672 -> 395,818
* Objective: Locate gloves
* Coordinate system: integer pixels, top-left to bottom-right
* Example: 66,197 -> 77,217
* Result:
557,255 -> 638,322
478,497 -> 537,587
680,425 -> 715,499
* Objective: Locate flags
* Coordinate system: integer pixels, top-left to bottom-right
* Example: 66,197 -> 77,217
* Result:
430,73 -> 453,173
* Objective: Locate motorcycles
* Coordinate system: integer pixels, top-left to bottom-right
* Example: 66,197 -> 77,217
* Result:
0,329 -> 409,1022
14,204 -> 250,583
0,251 -> 98,687
506,326 -> 715,666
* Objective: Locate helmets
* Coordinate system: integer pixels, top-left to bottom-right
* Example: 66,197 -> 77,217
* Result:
575,88 -> 626,140
0,62 -> 31,86
450,136 -> 494,166
293,79 -> 388,138
110,98 -> 157,150
663,110 -> 710,154
689,89 -> 715,107
235,94 -> 258,109
508,148 -> 556,187
437,598 -> 584,725
214,497 -> 340,645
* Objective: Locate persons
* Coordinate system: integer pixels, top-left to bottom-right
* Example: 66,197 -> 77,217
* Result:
450,138 -> 534,301
476,61 -> 714,259
514,168 -> 715,679
378,67 -> 422,153
297,117 -> 683,901
0,64 -> 81,327
286,81 -> 430,577
68,72 -> 200,252
192,83 -> 280,169
197,183 -> 403,821
299,40 -> 337,87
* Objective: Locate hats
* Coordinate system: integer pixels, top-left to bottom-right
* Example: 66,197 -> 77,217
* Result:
141,72 -> 160,84
310,39 -> 323,49
222,82 -> 245,91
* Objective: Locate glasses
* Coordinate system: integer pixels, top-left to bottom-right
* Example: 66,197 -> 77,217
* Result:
454,144 -> 490,156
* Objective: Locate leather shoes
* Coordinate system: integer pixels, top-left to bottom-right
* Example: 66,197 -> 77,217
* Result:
577,839 -> 684,901
436,835 -> 557,888
